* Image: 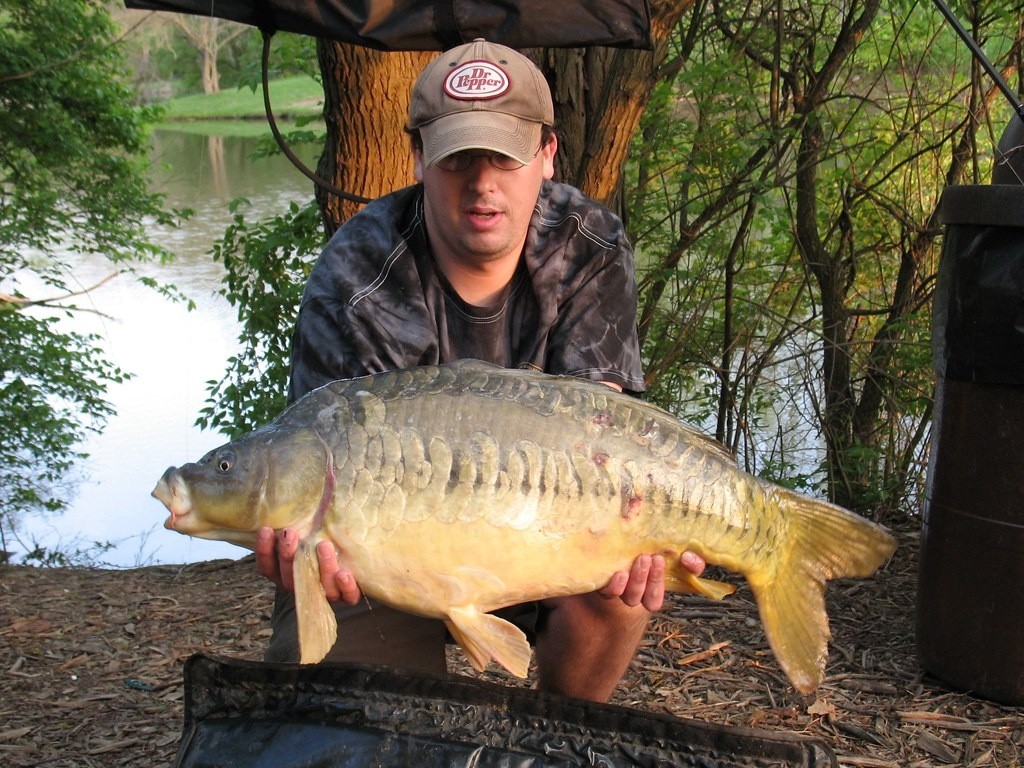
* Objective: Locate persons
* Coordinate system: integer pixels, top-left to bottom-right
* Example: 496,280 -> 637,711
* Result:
256,38 -> 705,704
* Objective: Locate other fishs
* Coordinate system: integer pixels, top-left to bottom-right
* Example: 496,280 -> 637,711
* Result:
148,358 -> 899,698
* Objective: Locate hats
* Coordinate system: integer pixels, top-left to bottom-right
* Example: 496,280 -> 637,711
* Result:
408,37 -> 555,166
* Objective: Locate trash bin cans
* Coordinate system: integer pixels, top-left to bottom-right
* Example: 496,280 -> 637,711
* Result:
907,182 -> 1023,712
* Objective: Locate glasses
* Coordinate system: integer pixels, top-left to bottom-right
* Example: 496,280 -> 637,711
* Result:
416,132 -> 551,173
